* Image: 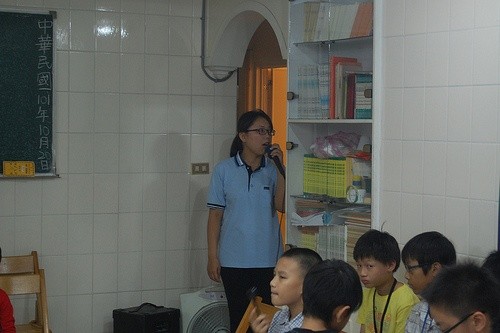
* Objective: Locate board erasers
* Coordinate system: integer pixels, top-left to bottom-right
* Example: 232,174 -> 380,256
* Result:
3,161 -> 35,177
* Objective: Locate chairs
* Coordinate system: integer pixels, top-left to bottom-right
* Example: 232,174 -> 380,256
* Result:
0,269 -> 48,333
234,296 -> 281,333
0,251 -> 52,333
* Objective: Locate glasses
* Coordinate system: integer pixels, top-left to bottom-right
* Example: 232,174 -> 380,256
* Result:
405,263 -> 430,273
247,127 -> 275,136
435,310 -> 486,333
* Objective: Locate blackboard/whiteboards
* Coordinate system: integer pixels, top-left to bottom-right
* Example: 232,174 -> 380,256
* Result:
0,5 -> 60,179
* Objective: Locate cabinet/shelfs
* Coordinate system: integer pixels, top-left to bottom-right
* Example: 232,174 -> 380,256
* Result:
285,35 -> 374,271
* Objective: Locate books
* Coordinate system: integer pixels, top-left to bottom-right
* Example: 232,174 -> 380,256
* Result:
304,2 -> 373,42
295,199 -> 370,269
296,55 -> 372,119
303,154 -> 371,198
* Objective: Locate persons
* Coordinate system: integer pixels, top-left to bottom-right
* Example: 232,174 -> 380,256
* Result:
249,248 -> 322,333
402,231 -> 500,333
206,109 -> 286,333
353,229 -> 421,333
286,259 -> 363,333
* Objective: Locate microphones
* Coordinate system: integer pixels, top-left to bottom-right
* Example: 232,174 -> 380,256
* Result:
265,145 -> 285,180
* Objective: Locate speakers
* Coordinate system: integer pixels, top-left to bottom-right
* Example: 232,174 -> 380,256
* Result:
112,303 -> 180,333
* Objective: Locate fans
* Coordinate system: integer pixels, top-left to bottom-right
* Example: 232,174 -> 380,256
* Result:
178,283 -> 230,333
